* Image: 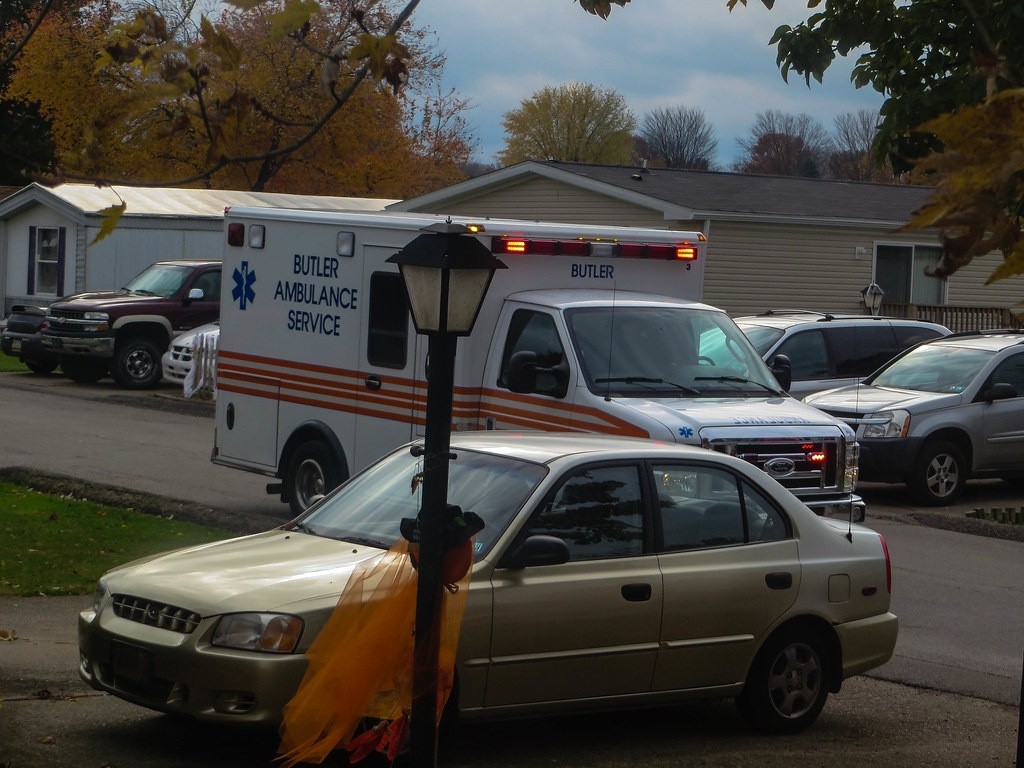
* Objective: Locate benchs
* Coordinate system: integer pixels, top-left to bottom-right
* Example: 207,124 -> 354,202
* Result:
659,493 -> 774,548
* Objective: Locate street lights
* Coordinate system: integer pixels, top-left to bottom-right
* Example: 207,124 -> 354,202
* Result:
382,214 -> 510,768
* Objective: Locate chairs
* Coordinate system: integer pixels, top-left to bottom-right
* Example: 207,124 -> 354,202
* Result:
487,474 -> 530,525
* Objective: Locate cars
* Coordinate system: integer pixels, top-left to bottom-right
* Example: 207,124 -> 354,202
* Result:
1,304 -> 59,377
78,429 -> 903,754
160,319 -> 221,392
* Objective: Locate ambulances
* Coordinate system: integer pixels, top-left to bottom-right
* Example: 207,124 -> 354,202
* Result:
209,202 -> 868,543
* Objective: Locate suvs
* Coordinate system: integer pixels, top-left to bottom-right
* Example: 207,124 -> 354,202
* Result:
682,307 -> 963,407
40,260 -> 224,390
800,328 -> 1024,508
